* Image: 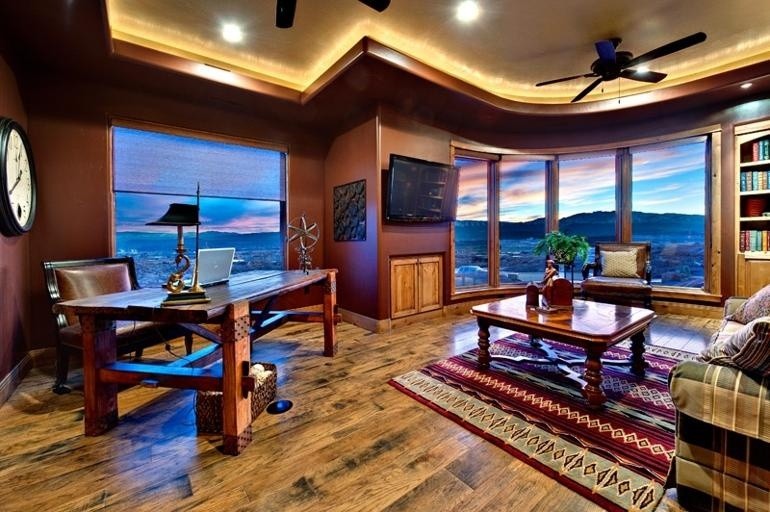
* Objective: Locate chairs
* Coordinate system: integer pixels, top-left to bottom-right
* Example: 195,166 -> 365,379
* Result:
668,296 -> 770,512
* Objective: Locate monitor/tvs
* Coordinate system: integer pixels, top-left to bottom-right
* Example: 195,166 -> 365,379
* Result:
383,152 -> 461,226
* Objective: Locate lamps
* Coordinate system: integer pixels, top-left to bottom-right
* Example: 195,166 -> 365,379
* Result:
145,203 -> 212,305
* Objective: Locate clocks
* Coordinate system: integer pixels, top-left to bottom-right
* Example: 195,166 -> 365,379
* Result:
0,118 -> 37,237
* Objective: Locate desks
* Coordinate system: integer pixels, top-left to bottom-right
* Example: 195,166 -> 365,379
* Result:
52,269 -> 340,456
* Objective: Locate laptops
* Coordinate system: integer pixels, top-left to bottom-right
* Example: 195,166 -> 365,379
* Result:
161,247 -> 236,287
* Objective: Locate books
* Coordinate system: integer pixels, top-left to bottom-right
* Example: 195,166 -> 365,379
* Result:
738,140 -> 770,251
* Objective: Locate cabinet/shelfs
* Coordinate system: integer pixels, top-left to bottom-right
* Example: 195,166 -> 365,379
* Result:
734,120 -> 770,298
391,255 -> 444,319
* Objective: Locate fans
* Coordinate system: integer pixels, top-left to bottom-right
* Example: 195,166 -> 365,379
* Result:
536,32 -> 707,104
275,0 -> 392,29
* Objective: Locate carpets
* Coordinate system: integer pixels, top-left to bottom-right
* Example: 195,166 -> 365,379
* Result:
385,332 -> 698,512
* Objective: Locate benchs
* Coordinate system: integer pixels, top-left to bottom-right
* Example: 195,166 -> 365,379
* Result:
40,255 -> 193,395
581,243 -> 652,309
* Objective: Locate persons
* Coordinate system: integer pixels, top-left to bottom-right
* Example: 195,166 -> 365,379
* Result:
539,258 -> 557,311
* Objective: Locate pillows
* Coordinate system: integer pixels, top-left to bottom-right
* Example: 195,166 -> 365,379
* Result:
696,285 -> 770,370
600,248 -> 641,278
